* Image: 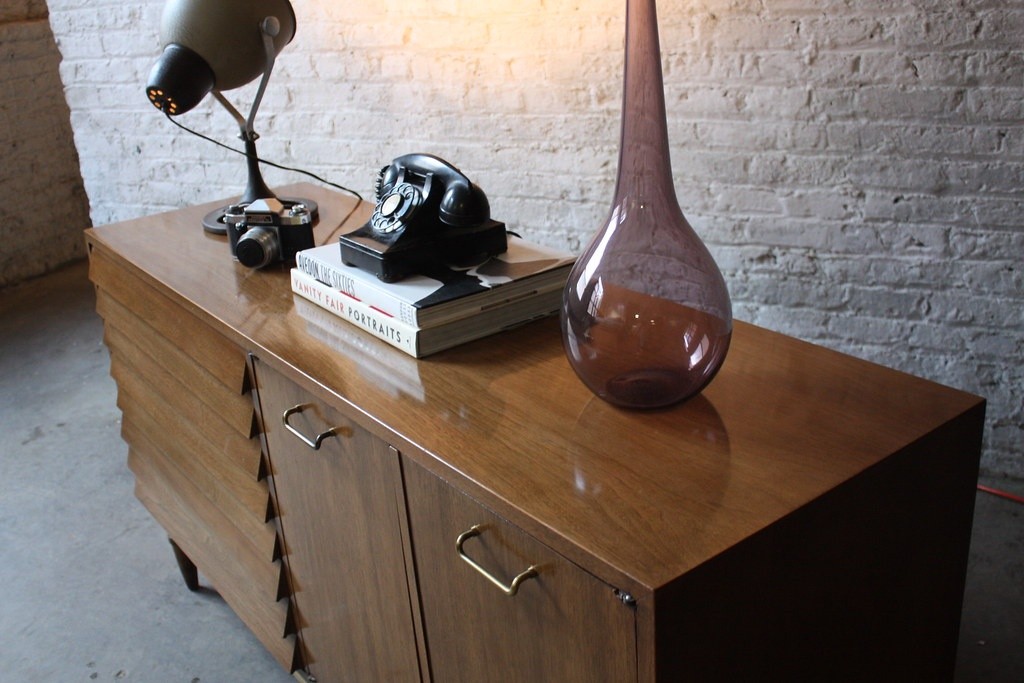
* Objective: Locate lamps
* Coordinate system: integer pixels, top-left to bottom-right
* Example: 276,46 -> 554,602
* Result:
145,0 -> 318,237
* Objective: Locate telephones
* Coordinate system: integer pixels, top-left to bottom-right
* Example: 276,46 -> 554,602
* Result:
338,152 -> 509,284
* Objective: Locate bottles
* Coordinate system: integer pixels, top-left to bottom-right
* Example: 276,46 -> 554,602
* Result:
559,0 -> 734,416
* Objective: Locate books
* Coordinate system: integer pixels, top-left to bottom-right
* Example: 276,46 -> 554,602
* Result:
289,232 -> 579,358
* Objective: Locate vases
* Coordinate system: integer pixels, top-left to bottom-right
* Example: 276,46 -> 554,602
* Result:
561,1 -> 732,412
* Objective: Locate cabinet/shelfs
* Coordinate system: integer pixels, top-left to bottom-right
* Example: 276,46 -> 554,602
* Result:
87,182 -> 987,683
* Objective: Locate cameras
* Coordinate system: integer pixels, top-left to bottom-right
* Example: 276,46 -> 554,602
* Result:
223,198 -> 316,268
